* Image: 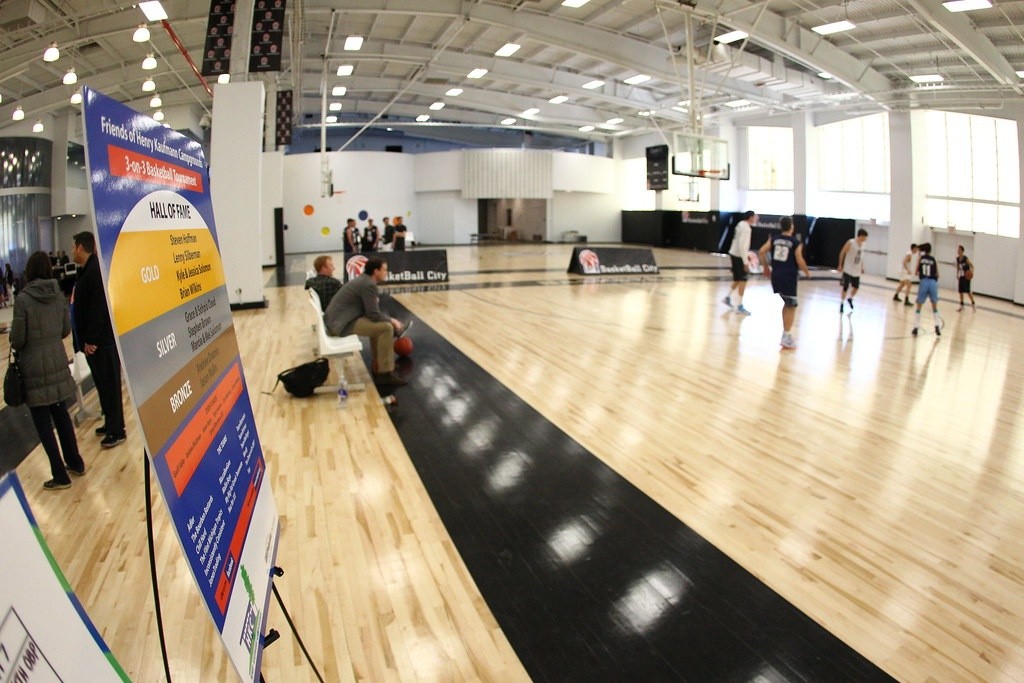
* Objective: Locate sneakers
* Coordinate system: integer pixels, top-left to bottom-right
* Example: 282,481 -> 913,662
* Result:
43,479 -> 71,490
780,332 -> 796,349
96,424 -> 125,435
101,429 -> 126,448
64,463 -> 86,475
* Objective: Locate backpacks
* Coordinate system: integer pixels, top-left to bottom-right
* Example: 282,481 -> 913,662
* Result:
278,358 -> 330,397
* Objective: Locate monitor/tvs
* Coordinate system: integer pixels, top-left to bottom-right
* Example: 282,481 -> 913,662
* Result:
65,263 -> 76,275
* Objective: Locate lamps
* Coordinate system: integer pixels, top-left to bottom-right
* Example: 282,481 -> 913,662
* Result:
10,2 -> 172,131
908,55 -> 945,83
810,2 -> 857,35
942,0 -> 995,14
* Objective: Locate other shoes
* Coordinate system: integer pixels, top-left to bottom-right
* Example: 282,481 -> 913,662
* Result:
723,297 -> 734,308
913,329 -> 917,334
905,301 -> 913,306
736,305 -> 750,316
936,327 -> 940,335
848,298 -> 853,308
894,295 -> 902,301
375,373 -> 408,386
395,320 -> 413,340
972,304 -> 976,312
840,304 -> 843,312
957,305 -> 964,311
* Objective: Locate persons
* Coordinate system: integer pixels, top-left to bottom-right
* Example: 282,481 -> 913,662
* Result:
382,217 -> 396,252
9,251 -> 86,489
69,231 -> 128,446
912,243 -> 941,335
4,263 -> 14,305
722,210 -> 756,315
395,217 -> 406,251
758,217 -> 810,350
304,255 -> 413,339
323,255 -> 407,388
12,273 -> 26,296
364,219 -> 379,250
0,268 -> 8,308
352,220 -> 362,254
49,251 -> 70,266
344,219 -> 357,284
837,228 -> 868,312
956,245 -> 977,312
893,243 -> 919,306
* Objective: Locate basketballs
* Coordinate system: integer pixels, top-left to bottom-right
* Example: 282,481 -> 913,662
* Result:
394,336 -> 414,357
965,270 -> 973,280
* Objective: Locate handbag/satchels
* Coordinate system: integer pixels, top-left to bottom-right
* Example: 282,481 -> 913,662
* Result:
4,340 -> 25,407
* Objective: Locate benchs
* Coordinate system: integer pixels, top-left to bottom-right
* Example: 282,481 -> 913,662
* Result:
306,269 -> 367,393
